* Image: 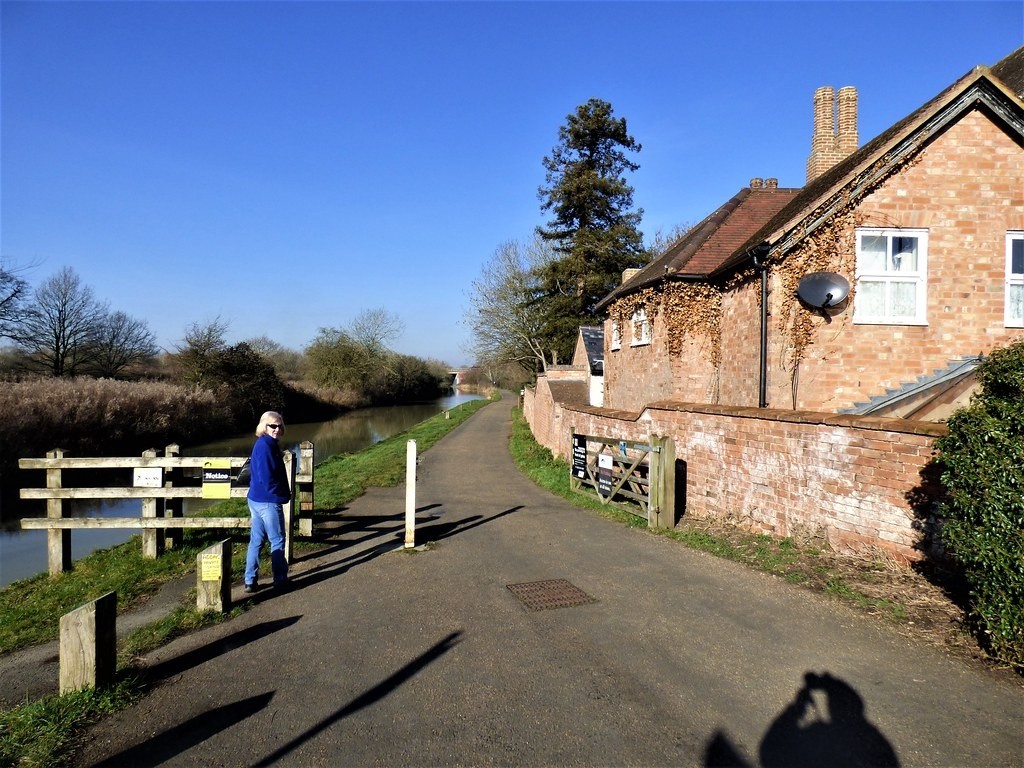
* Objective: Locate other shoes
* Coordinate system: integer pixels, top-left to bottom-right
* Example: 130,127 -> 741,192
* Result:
273,581 -> 298,590
245,582 -> 258,592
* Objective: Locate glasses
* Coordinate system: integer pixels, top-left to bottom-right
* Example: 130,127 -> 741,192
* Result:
267,424 -> 284,429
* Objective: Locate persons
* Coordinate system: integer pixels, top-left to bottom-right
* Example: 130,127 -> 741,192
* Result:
245,412 -> 293,594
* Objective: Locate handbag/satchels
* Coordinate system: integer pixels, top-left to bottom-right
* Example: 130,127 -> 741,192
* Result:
236,458 -> 251,485
